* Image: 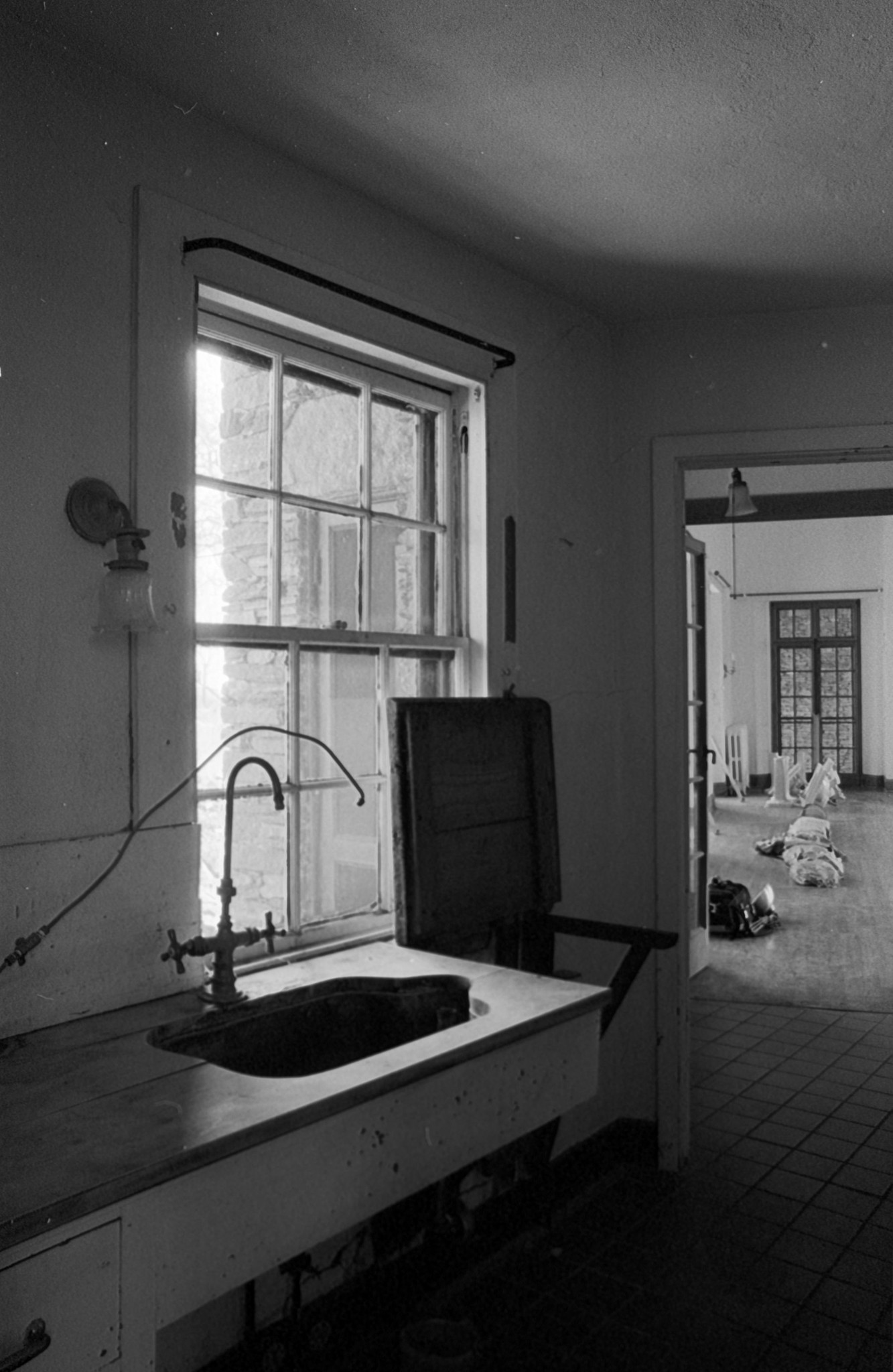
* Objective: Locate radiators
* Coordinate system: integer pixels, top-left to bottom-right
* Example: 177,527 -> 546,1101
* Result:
725,720 -> 753,795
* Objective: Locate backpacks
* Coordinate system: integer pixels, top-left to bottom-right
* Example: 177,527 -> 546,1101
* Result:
699,876 -> 754,936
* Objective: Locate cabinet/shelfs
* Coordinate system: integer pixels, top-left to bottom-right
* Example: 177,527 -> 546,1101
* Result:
0,1188 -> 156,1372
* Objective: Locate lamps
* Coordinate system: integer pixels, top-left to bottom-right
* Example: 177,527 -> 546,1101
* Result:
60,470 -> 168,634
722,465 -> 760,519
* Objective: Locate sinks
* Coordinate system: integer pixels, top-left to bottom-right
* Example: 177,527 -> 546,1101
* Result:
143,972 -> 492,1081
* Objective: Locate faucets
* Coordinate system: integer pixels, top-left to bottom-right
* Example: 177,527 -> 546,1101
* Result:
156,754 -> 287,1008
